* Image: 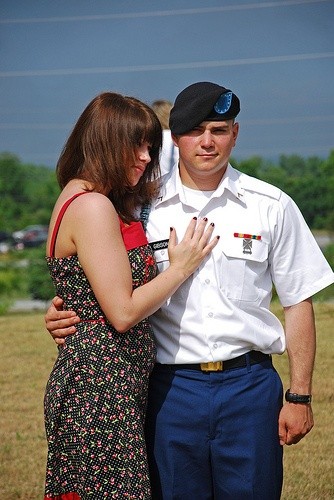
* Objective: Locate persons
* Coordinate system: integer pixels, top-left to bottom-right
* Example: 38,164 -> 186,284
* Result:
44,81 -> 334,500
44,92 -> 220,500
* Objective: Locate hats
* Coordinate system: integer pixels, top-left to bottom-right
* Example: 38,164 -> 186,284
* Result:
168,82 -> 241,136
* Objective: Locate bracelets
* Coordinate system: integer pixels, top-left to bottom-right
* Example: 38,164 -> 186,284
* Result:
285,388 -> 312,405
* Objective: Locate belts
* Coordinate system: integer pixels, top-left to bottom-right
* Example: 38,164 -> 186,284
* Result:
172,351 -> 270,372
